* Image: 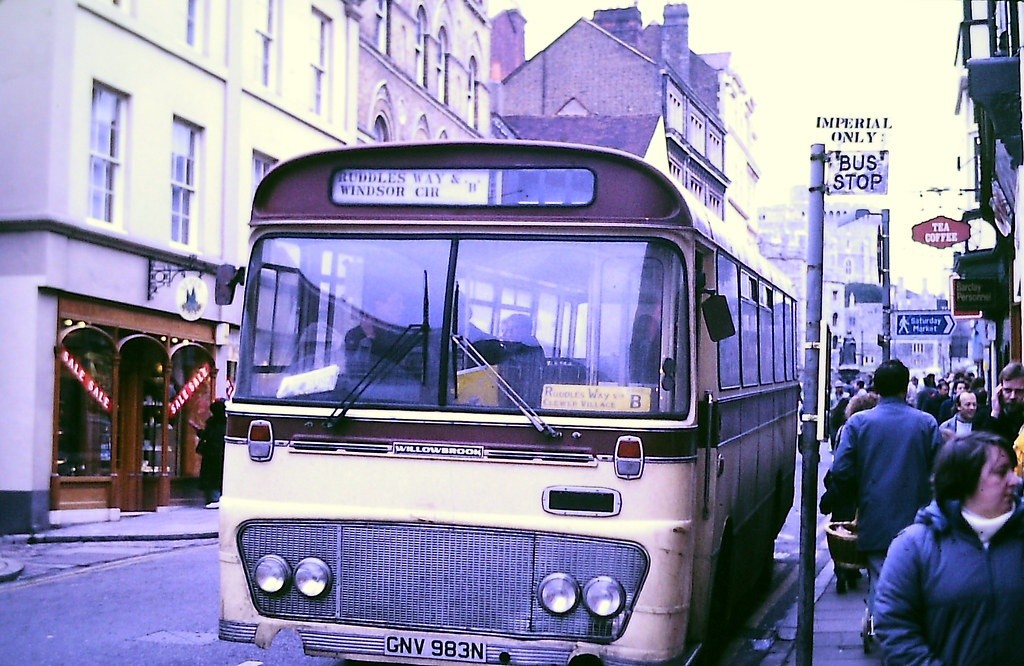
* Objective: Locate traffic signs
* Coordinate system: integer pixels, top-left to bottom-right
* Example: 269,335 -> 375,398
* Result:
890,309 -> 957,341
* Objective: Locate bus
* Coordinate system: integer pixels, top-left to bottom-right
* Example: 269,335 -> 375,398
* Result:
211,136 -> 805,666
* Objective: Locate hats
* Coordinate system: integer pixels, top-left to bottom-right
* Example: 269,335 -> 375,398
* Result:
209,402 -> 226,415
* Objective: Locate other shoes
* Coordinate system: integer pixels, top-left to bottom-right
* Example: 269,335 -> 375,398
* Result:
206,502 -> 219,508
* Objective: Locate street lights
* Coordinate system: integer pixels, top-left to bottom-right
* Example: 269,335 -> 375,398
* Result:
834,208 -> 892,362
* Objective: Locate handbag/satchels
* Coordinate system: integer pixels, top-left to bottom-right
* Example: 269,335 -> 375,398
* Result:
196,437 -> 206,453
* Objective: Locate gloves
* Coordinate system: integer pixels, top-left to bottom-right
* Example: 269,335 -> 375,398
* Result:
819,490 -> 835,515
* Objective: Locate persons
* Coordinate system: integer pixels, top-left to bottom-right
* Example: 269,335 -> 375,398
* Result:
500,311 -> 546,408
819,359 -> 944,613
830,380 -> 850,451
971,360 -> 1024,441
873,429 -> 1024,666
905,376 -> 919,408
343,273 -> 426,401
830,392 -> 879,594
854,380 -> 868,396
867,373 -> 873,386
938,390 -> 977,442
420,284 -> 522,407
188,396 -> 227,510
916,371 -> 987,419
